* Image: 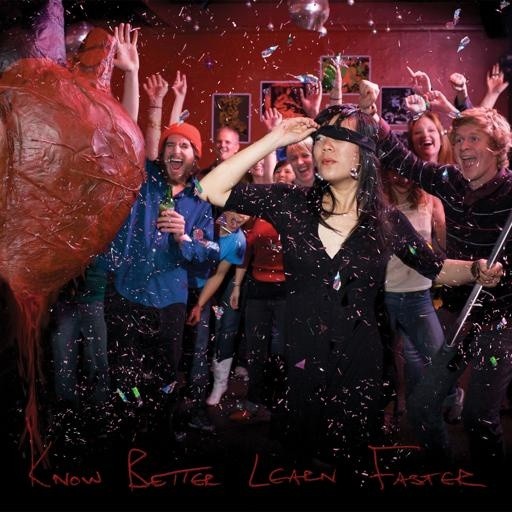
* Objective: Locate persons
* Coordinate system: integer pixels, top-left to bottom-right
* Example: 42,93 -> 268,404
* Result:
194,105 -> 502,464
360,63 -> 512,479
51,23 -> 342,398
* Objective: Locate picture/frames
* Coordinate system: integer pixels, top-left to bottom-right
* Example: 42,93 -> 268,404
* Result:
380,85 -> 415,131
260,79 -> 314,124
318,56 -> 371,95
208,92 -> 252,146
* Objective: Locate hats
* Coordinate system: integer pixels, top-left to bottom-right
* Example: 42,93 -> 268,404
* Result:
157,121 -> 201,159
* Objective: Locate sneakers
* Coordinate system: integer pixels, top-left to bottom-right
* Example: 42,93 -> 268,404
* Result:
447,386 -> 464,421
235,367 -> 248,378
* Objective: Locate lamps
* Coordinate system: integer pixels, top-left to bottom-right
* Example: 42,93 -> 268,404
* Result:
289,0 -> 330,31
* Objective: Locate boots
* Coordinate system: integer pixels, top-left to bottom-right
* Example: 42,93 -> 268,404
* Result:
205,355 -> 234,406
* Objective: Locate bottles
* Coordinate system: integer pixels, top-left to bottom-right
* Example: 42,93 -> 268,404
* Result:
159,183 -> 175,234
321,51 -> 340,93
